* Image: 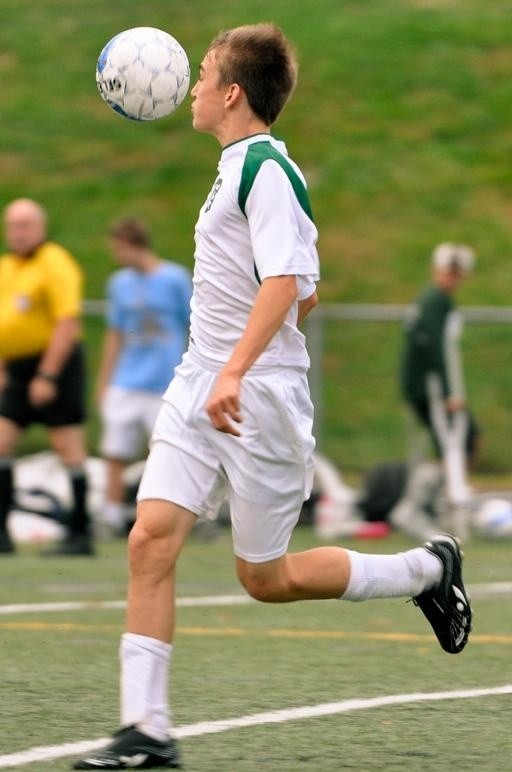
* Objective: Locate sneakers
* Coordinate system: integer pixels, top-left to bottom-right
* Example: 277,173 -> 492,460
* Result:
411,532 -> 473,656
73,726 -> 181,771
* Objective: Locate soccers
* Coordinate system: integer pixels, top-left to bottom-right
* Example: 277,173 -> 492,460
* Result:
96,27 -> 191,122
475,499 -> 512,536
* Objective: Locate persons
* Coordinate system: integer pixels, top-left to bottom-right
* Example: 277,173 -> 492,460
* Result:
92,214 -> 194,539
0,198 -> 99,554
71,23 -> 473,772
384,240 -> 479,546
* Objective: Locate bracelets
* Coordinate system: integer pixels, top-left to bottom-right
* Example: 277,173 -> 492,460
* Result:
35,370 -> 62,383
